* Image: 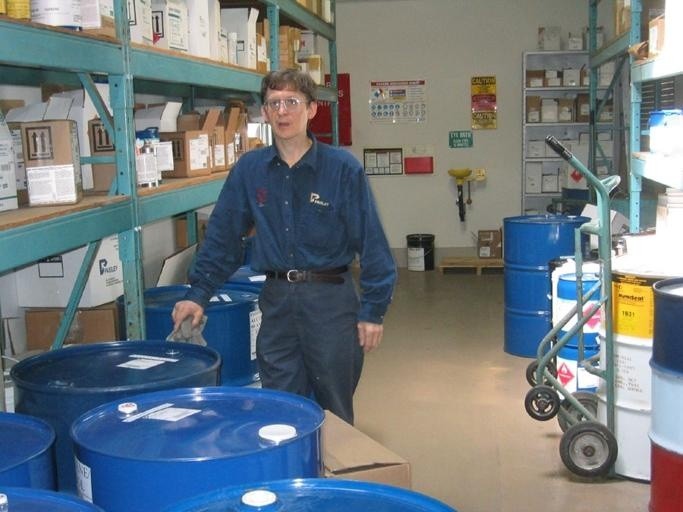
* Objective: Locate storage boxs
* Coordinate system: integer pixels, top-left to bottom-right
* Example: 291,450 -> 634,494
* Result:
309,406 -> 421,502
478,230 -> 502,260
89,0 -> 331,83
615,0 -> 665,66
525,60 -> 615,194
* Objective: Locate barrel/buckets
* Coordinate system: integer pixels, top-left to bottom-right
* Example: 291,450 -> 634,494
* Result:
10,339 -> 223,496
71,385 -> 326,510
158,477 -> 460,512
502,214 -> 593,361
547,256 -> 683,511
116,284 -> 263,388
1,411 -> 55,493
227,264 -> 267,288
1,486 -> 107,511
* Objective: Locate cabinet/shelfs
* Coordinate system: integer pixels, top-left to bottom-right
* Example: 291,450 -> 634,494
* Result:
585,1 -> 683,237
520,48 -> 621,214
0,3 -> 342,413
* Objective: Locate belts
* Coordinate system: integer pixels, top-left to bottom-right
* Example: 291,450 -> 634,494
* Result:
265,267 -> 348,284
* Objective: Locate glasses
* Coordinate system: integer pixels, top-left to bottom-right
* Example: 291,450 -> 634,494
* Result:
262,97 -> 308,110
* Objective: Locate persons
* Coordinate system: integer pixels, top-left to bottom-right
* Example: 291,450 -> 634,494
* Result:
172,68 -> 399,428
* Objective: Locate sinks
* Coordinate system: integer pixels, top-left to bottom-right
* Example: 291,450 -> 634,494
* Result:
447,169 -> 472,179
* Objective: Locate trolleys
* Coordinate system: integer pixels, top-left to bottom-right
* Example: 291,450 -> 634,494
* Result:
520,134 -> 621,478
